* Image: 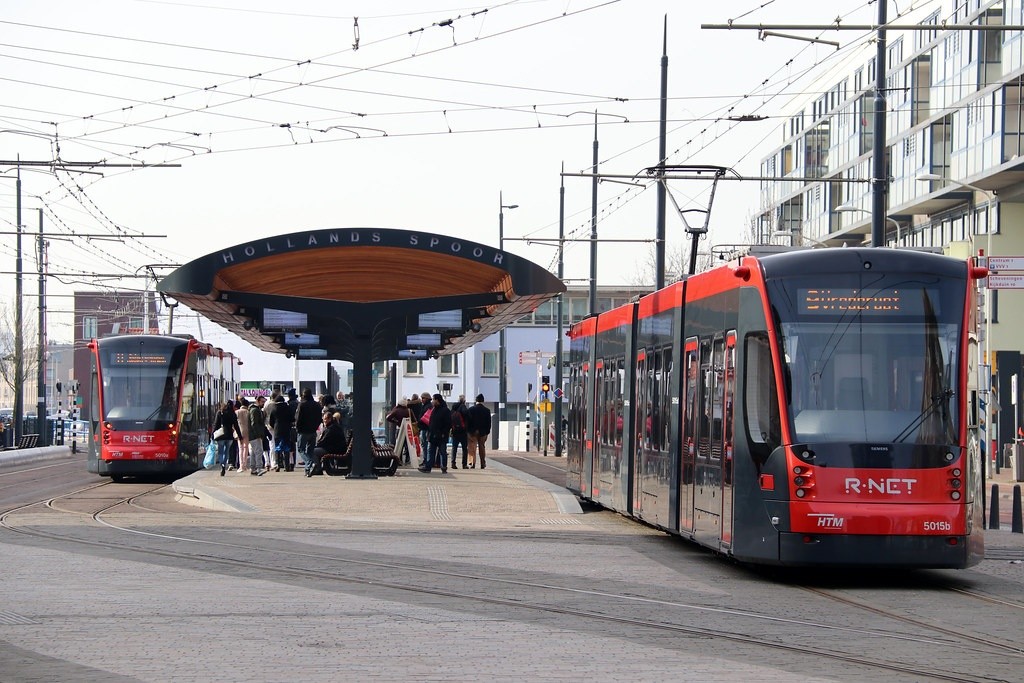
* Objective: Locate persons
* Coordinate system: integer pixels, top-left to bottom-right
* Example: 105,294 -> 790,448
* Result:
449,394 -> 470,469
263,387 -> 354,477
464,393 -> 492,469
210,400 -> 244,476
247,396 -> 273,476
385,392 -> 441,468
417,393 -> 450,474
228,397 -> 251,473
600,397 -> 667,452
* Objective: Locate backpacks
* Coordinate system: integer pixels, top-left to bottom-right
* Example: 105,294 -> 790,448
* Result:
452,410 -> 464,434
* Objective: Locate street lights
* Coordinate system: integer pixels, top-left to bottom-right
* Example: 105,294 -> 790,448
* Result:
499,205 -> 519,420
915,173 -> 992,480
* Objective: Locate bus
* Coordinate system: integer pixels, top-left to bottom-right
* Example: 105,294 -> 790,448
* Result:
566,248 -> 988,571
87,334 -> 243,482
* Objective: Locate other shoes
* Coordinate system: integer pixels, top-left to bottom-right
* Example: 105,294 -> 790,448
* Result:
418,462 -> 485,474
237,465 -> 294,476
221,465 -> 225,476
307,462 -> 317,477
228,465 -> 233,471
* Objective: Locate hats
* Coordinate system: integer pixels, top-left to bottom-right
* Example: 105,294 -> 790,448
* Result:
475,394 -> 485,402
398,396 -> 408,406
421,393 -> 432,399
274,396 -> 284,402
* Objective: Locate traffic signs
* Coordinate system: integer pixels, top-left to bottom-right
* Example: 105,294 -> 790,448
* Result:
519,351 -> 536,364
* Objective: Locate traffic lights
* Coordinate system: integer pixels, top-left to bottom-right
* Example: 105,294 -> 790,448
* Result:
542,384 -> 549,391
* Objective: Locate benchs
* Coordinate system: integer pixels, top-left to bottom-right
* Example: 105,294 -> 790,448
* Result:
370,430 -> 402,476
320,429 -> 353,476
4,433 -> 39,451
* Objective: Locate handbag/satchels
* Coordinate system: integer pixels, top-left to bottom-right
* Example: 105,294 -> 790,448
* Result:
421,407 -> 433,426
203,440 -> 216,469
213,427 -> 225,439
